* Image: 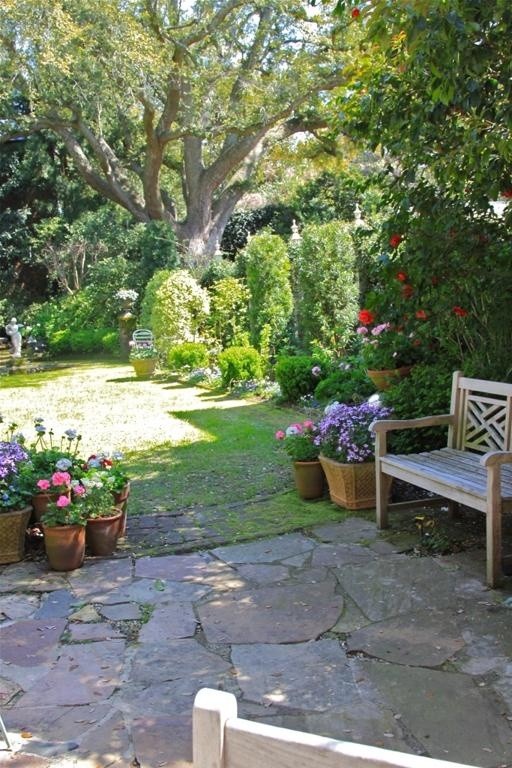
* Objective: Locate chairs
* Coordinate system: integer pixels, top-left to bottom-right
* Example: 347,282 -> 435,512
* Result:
131,329 -> 165,379
188,687 -> 481,768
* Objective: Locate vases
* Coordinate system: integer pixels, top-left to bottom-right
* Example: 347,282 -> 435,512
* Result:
290,459 -> 325,500
0,491 -> 129,569
318,449 -> 395,510
366,370 -> 411,390
121,299 -> 133,308
130,358 -> 157,374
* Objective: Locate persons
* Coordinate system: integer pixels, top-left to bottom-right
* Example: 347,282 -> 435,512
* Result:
5,318 -> 23,358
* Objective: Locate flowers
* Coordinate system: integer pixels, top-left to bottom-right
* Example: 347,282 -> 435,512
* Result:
345,318 -> 423,368
127,339 -> 158,358
112,288 -> 139,301
276,419 -> 320,460
0,415 -> 128,527
316,397 -> 396,461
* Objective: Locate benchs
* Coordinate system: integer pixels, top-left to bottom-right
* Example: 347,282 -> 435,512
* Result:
368,369 -> 512,590
